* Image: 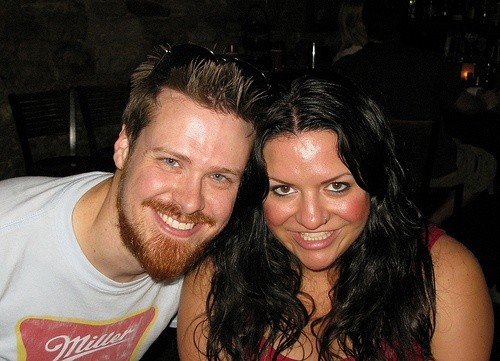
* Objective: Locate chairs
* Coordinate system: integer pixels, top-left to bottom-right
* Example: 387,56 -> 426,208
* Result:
6,86 -> 78,177
77,80 -> 133,172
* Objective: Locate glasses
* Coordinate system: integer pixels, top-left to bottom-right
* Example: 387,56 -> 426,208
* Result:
142,44 -> 272,100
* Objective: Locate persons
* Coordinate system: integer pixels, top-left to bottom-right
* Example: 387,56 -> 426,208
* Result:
0,38 -> 270,361
178,0 -> 500,361
